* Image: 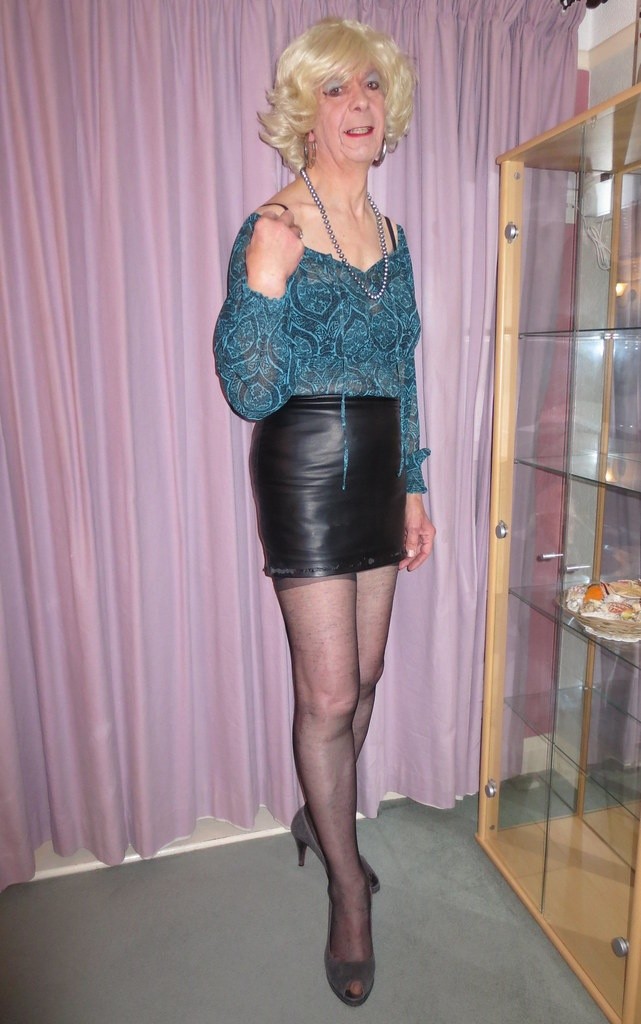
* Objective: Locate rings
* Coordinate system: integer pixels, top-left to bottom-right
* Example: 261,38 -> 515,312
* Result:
297,228 -> 304,239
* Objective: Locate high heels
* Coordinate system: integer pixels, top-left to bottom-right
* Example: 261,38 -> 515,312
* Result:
291,807 -> 379,893
324,888 -> 376,1008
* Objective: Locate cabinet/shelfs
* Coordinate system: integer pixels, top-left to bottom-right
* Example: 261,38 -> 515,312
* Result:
475,81 -> 641,1024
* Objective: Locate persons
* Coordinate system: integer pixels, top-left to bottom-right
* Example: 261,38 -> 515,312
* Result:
211,10 -> 438,1009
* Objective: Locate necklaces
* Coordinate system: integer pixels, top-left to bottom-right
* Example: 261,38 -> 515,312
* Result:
300,168 -> 390,300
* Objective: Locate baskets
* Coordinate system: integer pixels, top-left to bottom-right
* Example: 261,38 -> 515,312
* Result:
554,590 -> 641,640
